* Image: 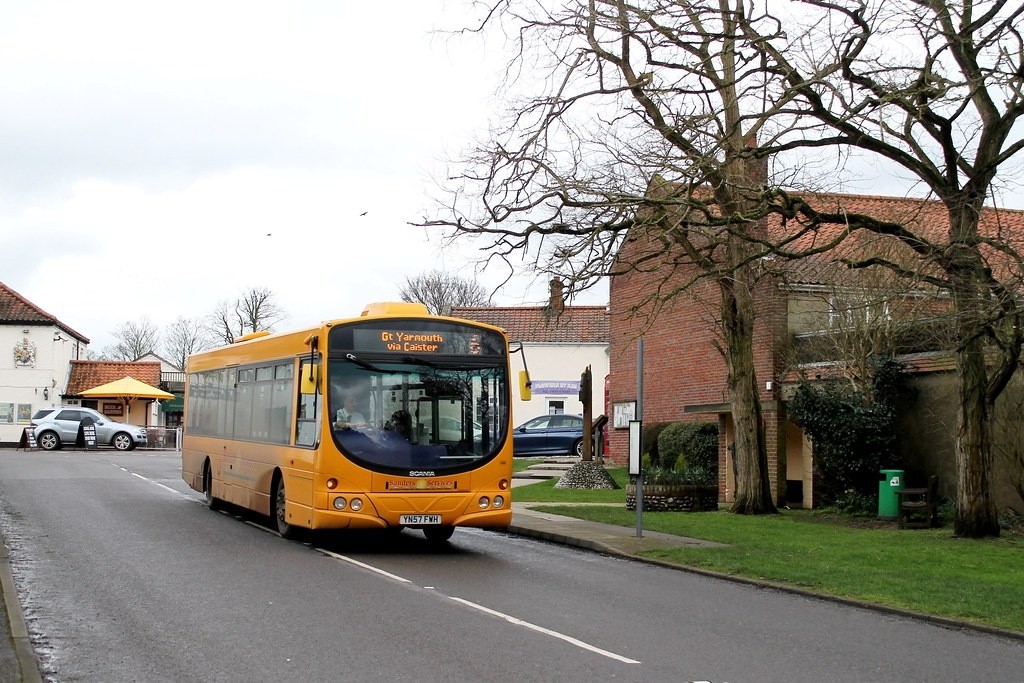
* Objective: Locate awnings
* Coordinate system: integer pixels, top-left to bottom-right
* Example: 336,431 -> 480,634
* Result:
161,393 -> 184,412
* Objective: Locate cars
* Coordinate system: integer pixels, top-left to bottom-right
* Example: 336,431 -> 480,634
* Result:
29,407 -> 149,451
415,414 -> 494,444
473,415 -> 595,458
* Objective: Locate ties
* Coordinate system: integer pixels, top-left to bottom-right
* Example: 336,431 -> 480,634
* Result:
347,415 -> 352,423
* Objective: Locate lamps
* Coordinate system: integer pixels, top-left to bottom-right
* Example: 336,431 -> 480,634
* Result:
44,387 -> 48,400
54,336 -> 68,344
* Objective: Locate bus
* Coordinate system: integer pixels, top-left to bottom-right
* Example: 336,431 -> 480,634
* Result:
183,302 -> 533,539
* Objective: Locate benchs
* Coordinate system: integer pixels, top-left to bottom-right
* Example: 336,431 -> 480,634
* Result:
146,429 -> 164,448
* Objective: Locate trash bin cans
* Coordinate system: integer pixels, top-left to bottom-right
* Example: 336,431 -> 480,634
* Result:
878,470 -> 905,521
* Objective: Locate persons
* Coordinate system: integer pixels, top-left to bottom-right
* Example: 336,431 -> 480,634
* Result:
337,392 -> 368,429
173,423 -> 183,448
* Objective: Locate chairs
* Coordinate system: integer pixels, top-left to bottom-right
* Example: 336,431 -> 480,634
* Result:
895,475 -> 939,529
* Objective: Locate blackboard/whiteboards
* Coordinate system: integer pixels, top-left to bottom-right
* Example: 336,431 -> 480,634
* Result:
20,427 -> 39,448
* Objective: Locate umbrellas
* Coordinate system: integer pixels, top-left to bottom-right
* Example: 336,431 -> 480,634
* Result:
77,375 -> 175,423
